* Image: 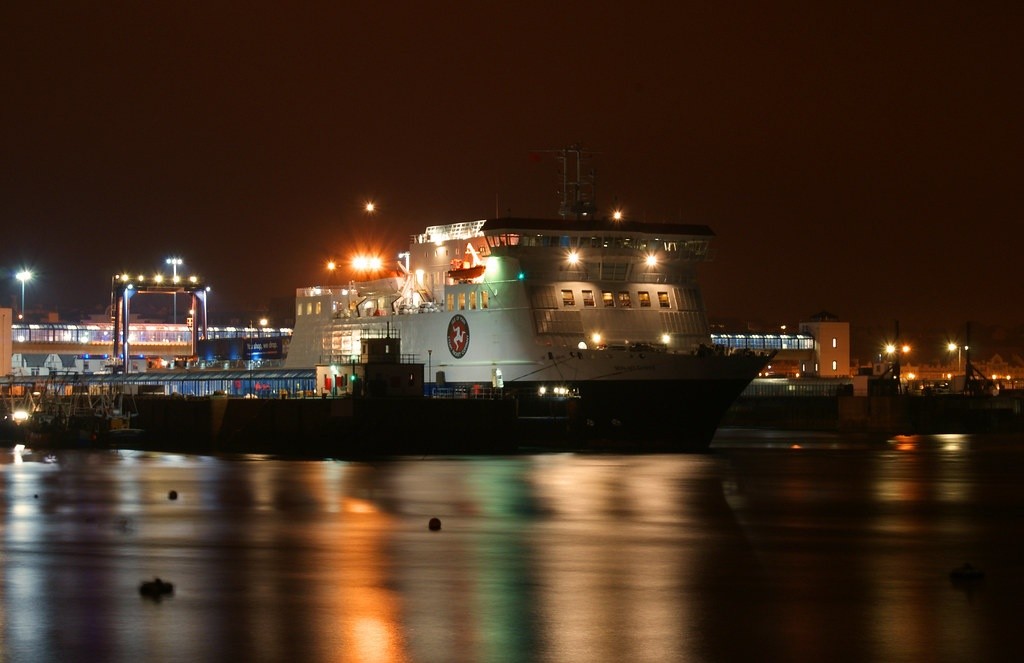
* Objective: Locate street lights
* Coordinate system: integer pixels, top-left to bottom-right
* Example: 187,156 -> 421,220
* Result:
949,345 -> 961,371
249,319 -> 268,399
17,273 -> 29,323
167,258 -> 182,324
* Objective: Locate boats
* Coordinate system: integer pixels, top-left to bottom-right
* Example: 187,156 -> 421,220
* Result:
23,370 -> 146,449
282,145 -> 779,452
